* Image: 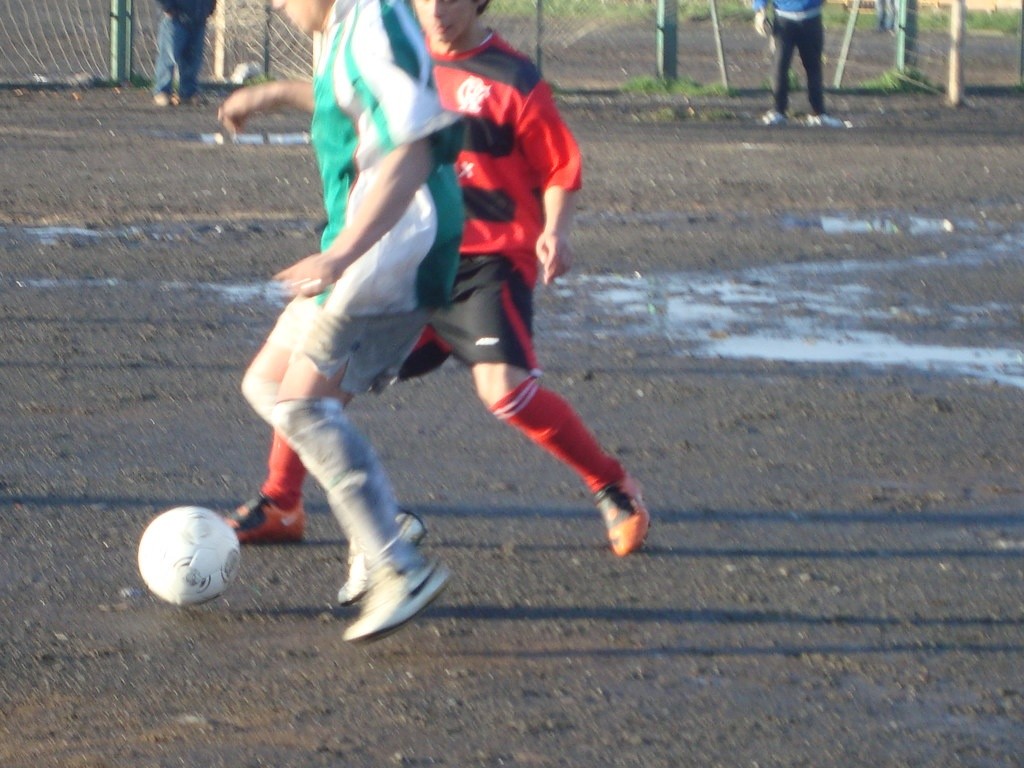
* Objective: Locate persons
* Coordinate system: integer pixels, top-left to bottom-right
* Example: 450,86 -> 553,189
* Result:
752,0 -> 845,129
151,0 -> 216,108
213,0 -> 472,642
224,0 -> 649,559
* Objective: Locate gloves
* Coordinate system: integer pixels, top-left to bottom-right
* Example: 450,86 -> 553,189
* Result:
754,11 -> 774,38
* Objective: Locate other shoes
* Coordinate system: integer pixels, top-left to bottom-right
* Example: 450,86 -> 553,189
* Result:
807,113 -> 844,128
172,93 -> 195,106
155,91 -> 169,106
758,111 -> 789,125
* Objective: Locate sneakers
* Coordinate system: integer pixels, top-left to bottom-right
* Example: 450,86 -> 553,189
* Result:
226,496 -> 307,546
337,515 -> 427,604
341,561 -> 454,645
597,468 -> 649,556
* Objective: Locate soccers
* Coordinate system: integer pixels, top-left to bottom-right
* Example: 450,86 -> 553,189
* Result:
135,508 -> 239,608
